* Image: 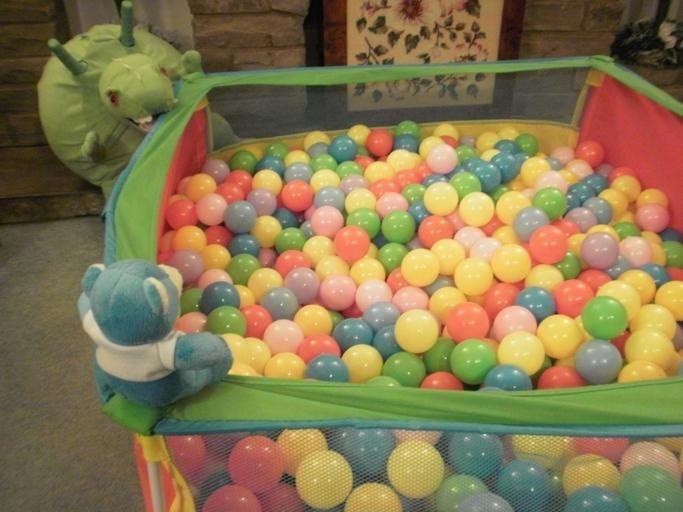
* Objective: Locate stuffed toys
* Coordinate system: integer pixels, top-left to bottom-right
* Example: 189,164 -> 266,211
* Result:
36,0 -> 241,207
75,256 -> 233,412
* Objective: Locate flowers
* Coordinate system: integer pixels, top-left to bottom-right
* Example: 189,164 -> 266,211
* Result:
349,1 -> 491,105
609,18 -> 682,69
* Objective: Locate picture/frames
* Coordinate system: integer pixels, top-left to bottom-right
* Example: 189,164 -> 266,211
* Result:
301,0 -> 526,129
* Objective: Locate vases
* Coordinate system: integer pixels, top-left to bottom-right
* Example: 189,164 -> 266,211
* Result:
632,68 -> 682,88
